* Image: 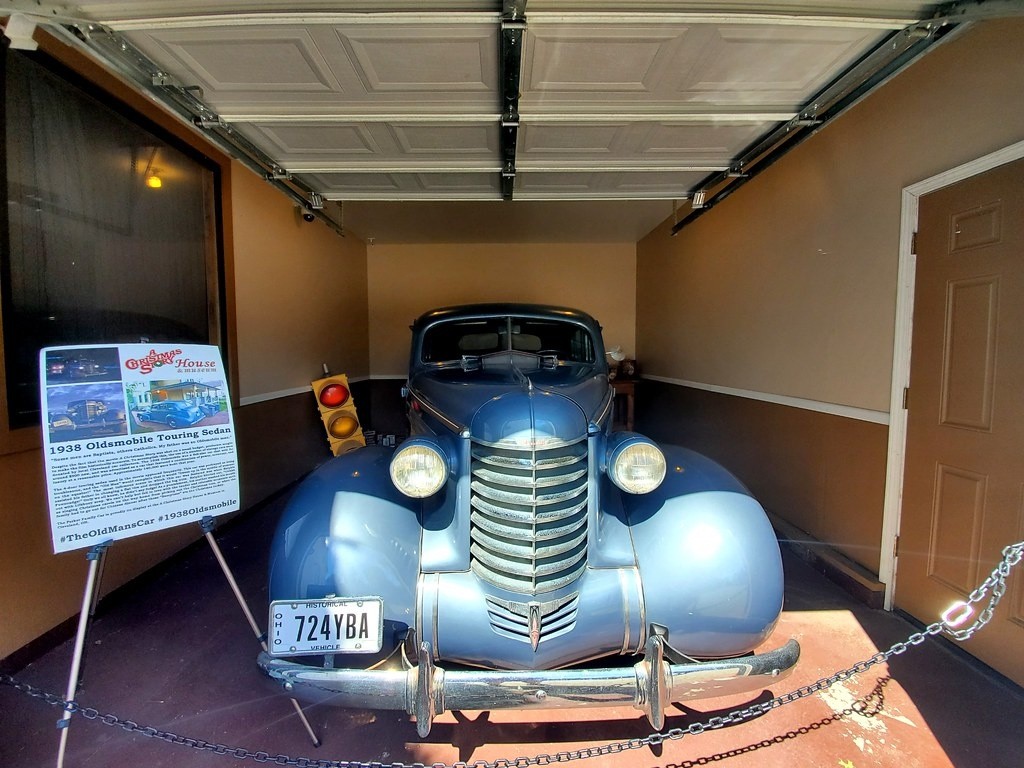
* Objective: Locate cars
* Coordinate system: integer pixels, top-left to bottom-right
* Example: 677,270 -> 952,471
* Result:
136,394 -> 220,430
253,302 -> 800,739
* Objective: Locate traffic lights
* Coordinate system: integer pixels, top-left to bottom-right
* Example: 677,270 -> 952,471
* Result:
310,373 -> 366,458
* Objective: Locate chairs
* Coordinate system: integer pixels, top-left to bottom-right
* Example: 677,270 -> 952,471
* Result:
459,333 -> 499,353
501,333 -> 542,351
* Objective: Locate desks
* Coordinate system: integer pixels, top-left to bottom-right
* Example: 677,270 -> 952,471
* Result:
612,379 -> 642,432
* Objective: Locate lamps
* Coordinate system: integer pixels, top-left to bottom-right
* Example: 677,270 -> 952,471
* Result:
497,12 -> 529,30
501,107 -> 521,128
501,162 -> 516,177
146,169 -> 162,188
